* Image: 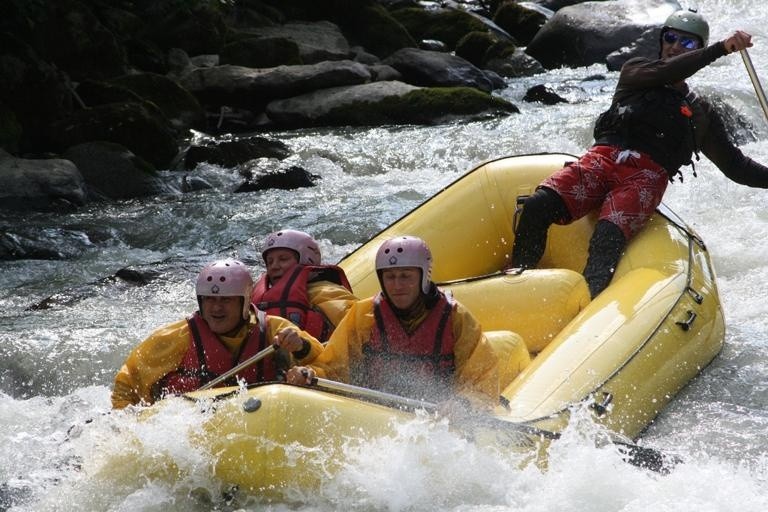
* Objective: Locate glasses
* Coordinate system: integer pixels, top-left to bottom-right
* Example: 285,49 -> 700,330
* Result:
664,31 -> 696,50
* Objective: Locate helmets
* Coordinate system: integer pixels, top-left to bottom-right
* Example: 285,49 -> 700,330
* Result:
262,229 -> 321,265
661,6 -> 710,47
196,258 -> 252,321
375,235 -> 432,295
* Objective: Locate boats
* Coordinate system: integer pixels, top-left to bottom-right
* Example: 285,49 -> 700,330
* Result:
81,153 -> 722,512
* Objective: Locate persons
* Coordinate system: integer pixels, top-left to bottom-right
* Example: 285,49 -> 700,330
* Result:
284,234 -> 503,433
249,230 -> 359,344
509,9 -> 767,302
111,257 -> 326,412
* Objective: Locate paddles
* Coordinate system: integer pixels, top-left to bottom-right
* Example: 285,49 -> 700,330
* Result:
301,375 -> 682,478
